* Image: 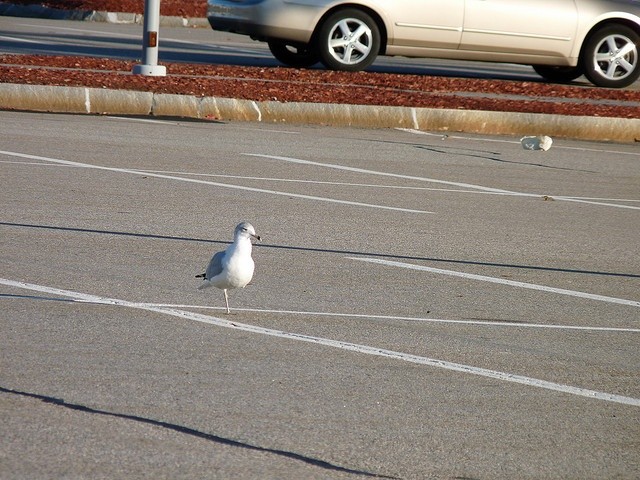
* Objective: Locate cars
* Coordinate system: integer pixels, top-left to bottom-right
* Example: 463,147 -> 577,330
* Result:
206,0 -> 640,88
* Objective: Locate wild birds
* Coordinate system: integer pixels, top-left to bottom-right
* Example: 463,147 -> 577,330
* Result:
194,221 -> 262,316
